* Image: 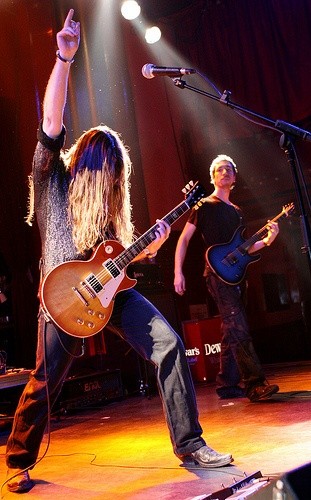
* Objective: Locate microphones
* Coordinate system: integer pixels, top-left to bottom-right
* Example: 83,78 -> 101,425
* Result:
141,63 -> 196,79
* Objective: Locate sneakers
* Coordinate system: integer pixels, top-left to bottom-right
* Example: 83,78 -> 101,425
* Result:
247,384 -> 279,403
219,386 -> 249,399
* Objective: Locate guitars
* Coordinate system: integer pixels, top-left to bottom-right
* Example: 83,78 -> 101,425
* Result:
206,203 -> 299,286
41,179 -> 206,338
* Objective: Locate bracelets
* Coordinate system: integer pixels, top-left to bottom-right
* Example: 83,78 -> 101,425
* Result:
262,238 -> 270,246
144,245 -> 157,259
55,50 -> 74,64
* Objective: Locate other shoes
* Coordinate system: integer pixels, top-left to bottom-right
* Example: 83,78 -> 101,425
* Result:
176,446 -> 233,468
6,469 -> 31,492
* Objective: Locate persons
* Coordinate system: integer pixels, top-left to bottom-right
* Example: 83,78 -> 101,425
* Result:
5,9 -> 235,491
174,154 -> 279,403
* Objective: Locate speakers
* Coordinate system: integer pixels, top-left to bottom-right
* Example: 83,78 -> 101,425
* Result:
122,291 -> 179,395
180,313 -> 222,383
